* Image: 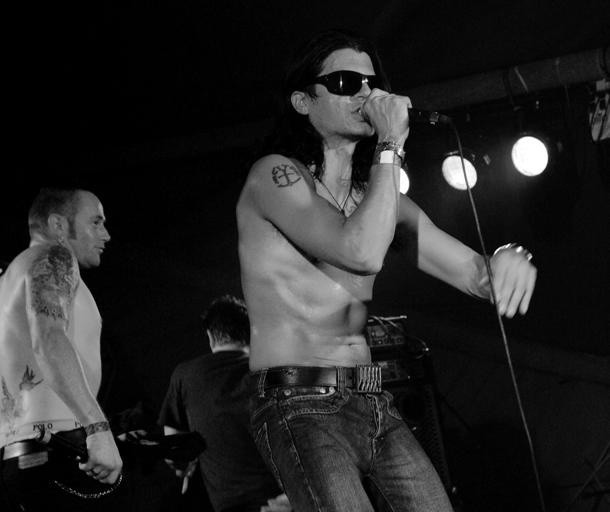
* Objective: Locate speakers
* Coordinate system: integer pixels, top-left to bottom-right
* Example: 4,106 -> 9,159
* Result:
370,352 -> 457,512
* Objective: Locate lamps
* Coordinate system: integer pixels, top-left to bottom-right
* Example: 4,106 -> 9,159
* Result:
511,128 -> 572,177
442,144 -> 481,191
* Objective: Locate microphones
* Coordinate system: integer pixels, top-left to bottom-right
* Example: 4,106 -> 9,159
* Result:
408,109 -> 448,127
37,430 -> 89,463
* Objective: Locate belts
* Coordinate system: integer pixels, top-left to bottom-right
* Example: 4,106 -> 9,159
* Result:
3,426 -> 86,463
251,365 -> 382,395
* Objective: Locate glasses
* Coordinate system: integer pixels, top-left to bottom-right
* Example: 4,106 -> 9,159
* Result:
304,69 -> 383,97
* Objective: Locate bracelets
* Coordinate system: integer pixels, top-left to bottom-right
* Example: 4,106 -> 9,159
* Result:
84,420 -> 111,436
373,140 -> 407,167
492,242 -> 537,264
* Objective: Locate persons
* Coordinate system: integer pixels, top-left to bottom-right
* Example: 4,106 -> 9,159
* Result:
230,28 -> 545,511
153,293 -> 291,511
0,181 -> 127,509
